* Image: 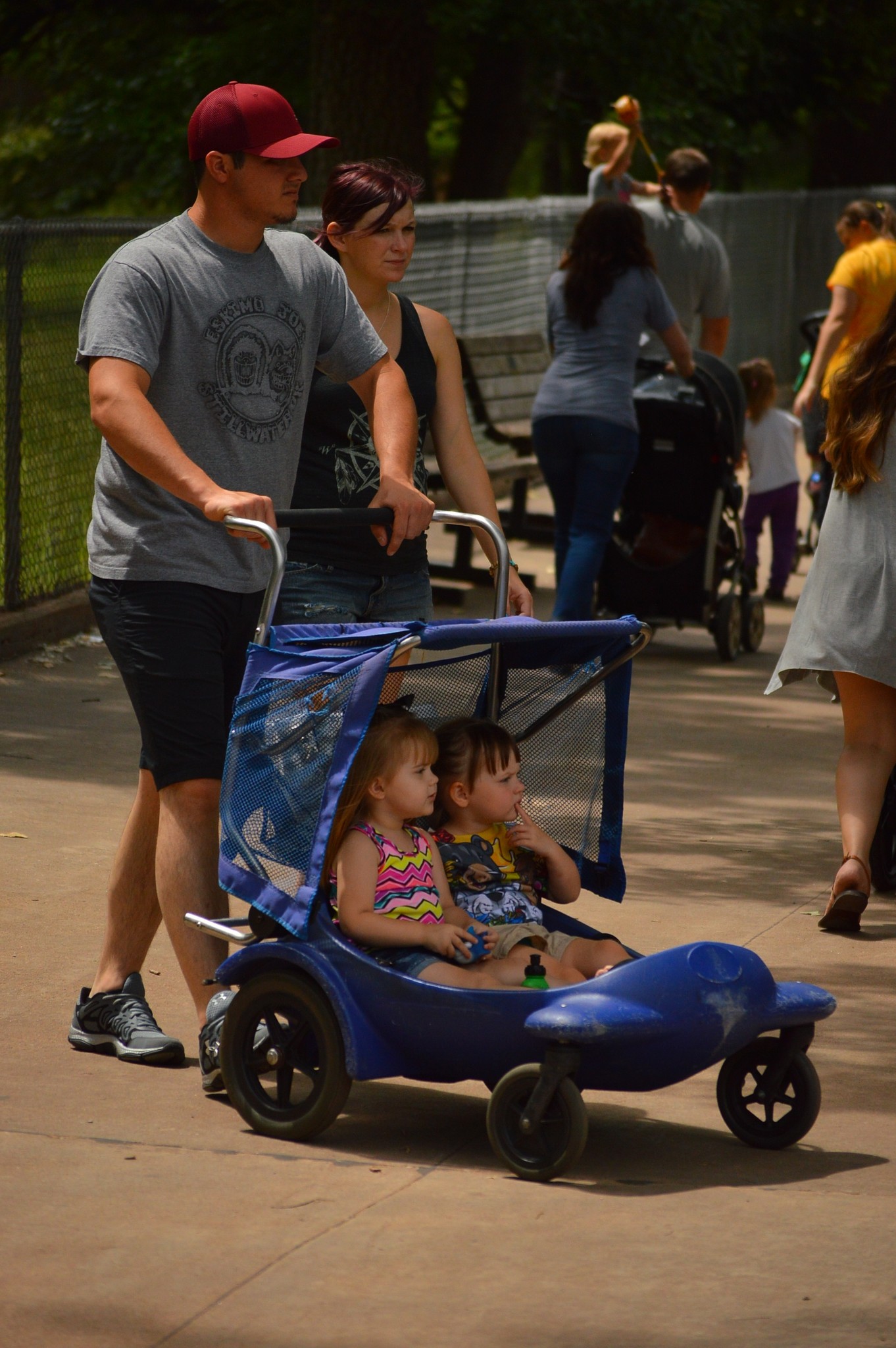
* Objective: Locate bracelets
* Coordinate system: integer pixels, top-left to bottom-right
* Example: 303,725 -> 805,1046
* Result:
488,558 -> 519,578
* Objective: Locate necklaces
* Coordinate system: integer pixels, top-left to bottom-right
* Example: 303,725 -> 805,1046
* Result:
377,289 -> 391,336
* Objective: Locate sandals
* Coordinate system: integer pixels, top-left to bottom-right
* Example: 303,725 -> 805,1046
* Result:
816,853 -> 873,931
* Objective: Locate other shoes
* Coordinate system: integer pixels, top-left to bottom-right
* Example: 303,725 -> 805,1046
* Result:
551,663 -> 576,680
740,571 -> 758,591
764,587 -> 784,601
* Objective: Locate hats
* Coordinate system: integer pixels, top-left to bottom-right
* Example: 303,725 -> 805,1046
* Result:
187,79 -> 341,163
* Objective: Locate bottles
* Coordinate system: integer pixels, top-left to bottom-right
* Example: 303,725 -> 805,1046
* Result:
520,953 -> 552,990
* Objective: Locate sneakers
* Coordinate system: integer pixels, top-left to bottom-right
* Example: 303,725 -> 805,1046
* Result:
197,987 -> 294,1093
68,970 -> 185,1064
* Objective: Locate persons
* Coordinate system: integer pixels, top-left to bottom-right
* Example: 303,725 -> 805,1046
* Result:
265,160 -> 533,777
737,356 -> 803,604
66,78 -> 437,1099
515,197 -> 699,677
421,715 -> 635,987
585,119 -> 675,213
762,200 -> 895,930
638,146 -> 733,366
630,416 -> 731,570
322,702 -> 571,991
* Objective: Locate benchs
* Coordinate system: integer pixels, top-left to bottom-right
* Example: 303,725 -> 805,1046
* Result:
425,328 -> 552,604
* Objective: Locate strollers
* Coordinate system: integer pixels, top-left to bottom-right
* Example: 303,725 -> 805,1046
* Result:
174,501 -> 836,1179
580,349 -> 768,663
